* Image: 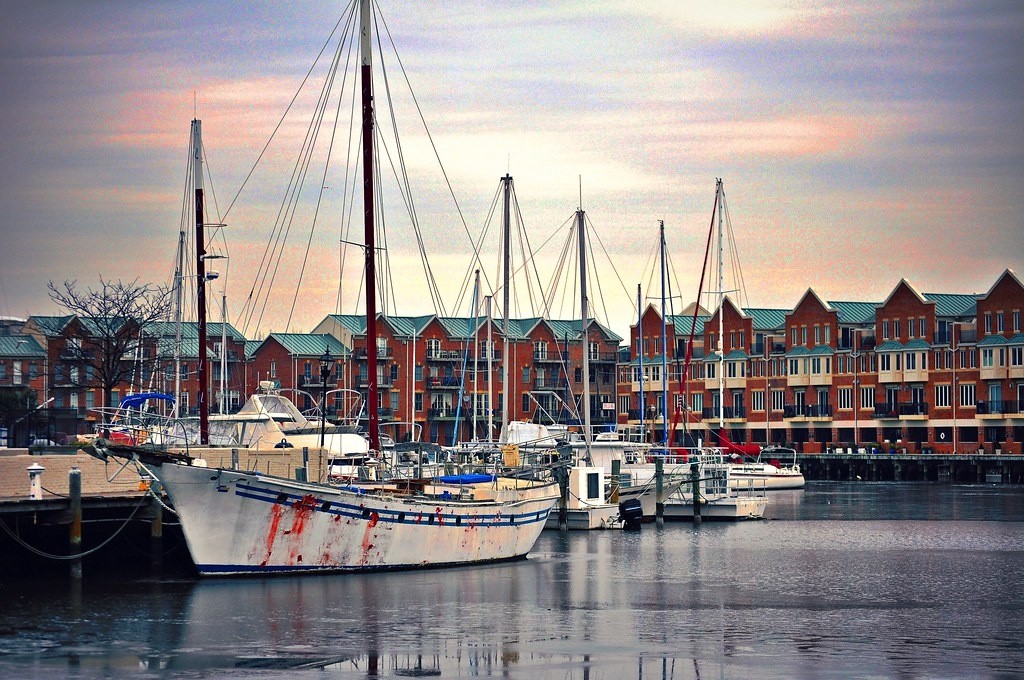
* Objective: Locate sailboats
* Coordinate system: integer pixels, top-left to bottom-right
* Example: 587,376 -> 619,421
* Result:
74,0 -> 814,580
